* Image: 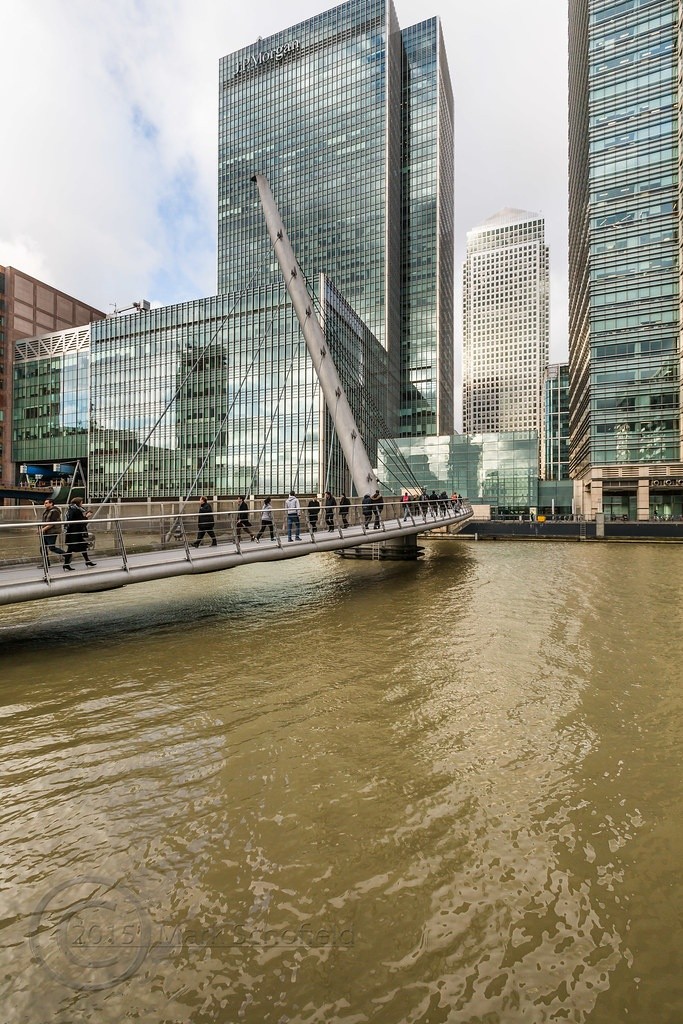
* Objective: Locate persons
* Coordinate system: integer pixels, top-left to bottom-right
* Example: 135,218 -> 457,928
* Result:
324,492 -> 337,532
307,496 -> 320,533
232,495 -> 255,544
36,498 -> 67,568
191,496 -> 217,548
400,490 -> 462,521
362,490 -> 384,529
339,493 -> 351,529
62,497 -> 97,571
255,497 -> 276,544
284,491 -> 302,542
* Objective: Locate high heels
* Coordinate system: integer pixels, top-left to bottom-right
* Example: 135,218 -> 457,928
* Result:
86,563 -> 96,567
63,564 -> 75,571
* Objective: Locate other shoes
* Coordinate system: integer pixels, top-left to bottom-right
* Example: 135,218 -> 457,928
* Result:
288,539 -> 293,542
38,565 -> 44,568
271,538 -> 276,541
251,536 -> 255,541
295,537 -> 302,540
191,543 -> 198,548
257,540 -> 260,543
209,543 -> 217,547
